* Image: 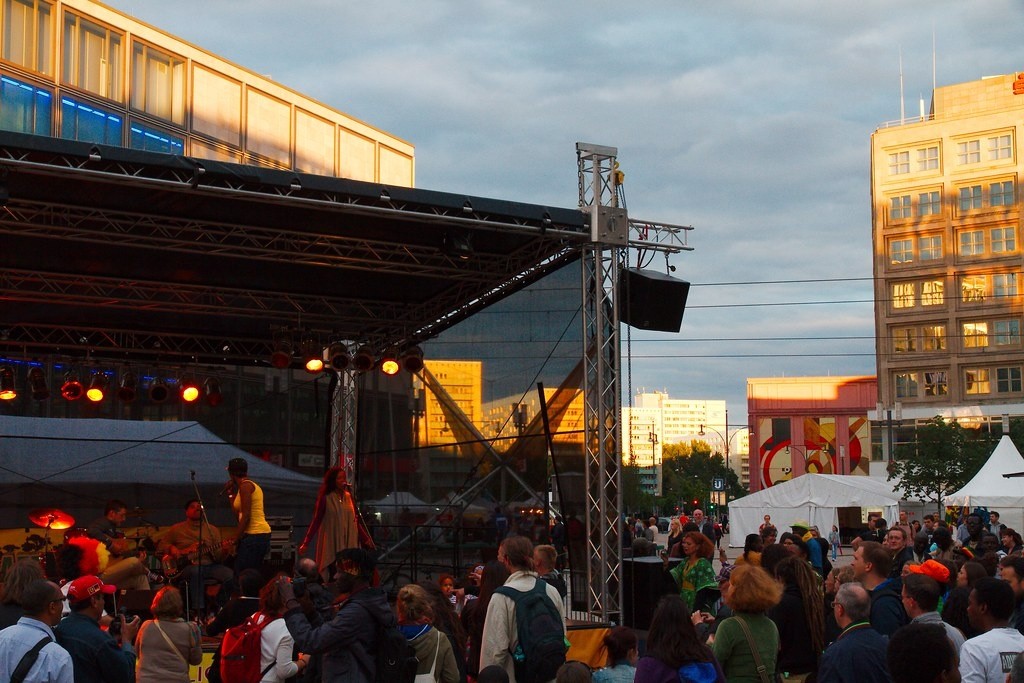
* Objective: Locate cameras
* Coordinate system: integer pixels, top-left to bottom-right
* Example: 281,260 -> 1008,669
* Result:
291,578 -> 306,598
661,549 -> 668,558
109,606 -> 135,635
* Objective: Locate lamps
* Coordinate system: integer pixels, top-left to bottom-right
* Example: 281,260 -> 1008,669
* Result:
0,329 -> 431,407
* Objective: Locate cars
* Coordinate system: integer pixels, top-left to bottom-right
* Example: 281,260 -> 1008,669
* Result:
385,507 -> 554,538
658,517 -> 672,531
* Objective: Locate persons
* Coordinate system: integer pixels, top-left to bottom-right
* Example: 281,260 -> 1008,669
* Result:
0,457 -> 1024,683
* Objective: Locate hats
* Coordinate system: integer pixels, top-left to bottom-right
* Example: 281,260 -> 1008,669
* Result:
909,559 -> 950,583
789,519 -> 809,529
67,574 -> 117,603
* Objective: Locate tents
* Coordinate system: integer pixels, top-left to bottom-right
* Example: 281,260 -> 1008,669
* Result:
0,416 -> 490,524
728,433 -> 1024,549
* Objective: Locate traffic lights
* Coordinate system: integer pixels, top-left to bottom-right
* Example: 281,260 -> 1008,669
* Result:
676,506 -> 679,510
694,500 -> 698,508
710,504 -> 714,510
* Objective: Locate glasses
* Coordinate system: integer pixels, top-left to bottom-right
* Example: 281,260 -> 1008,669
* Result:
49,595 -> 66,601
830,602 -> 840,608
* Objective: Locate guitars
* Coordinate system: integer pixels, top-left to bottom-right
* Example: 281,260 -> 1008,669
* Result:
64,528 -> 155,574
160,538 -> 238,579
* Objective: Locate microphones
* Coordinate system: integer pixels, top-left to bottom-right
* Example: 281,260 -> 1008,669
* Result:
343,483 -> 352,486
220,485 -> 230,496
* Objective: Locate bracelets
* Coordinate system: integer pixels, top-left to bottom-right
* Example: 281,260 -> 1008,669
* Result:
230,540 -> 238,544
298,658 -> 307,666
229,494 -> 234,497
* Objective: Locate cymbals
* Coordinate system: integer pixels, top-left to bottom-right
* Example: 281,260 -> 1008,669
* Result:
27,508 -> 76,530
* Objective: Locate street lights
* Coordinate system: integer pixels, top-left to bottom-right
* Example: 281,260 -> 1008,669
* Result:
648,433 -> 658,510
698,426 -> 756,515
675,469 -> 681,516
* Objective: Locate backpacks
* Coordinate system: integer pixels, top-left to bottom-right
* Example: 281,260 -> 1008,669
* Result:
493,579 -> 566,683
220,610 -> 283,683
339,599 -> 420,683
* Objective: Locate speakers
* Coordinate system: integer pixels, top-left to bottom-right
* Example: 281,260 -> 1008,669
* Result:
206,596 -> 263,637
622,556 -> 684,631
112,589 -> 159,630
617,268 -> 690,333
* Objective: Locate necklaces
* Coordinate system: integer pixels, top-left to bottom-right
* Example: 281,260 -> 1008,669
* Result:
337,495 -> 342,503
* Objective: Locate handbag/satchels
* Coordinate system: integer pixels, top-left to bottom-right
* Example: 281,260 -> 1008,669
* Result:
359,521 -> 375,551
